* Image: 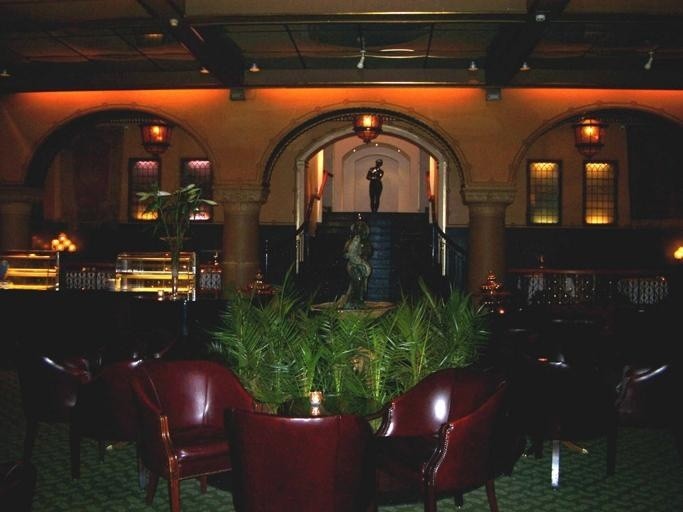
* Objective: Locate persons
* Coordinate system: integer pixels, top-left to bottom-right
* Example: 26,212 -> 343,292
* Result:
366,158 -> 383,212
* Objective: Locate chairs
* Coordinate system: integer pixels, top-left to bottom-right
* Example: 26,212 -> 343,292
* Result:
372,367 -> 517,511
8,330 -> 107,485
222,407 -> 380,512
479,295 -> 623,487
128,359 -> 264,510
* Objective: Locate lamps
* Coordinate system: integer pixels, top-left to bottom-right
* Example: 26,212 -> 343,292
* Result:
570,117 -> 610,161
352,113 -> 384,144
138,119 -> 170,158
